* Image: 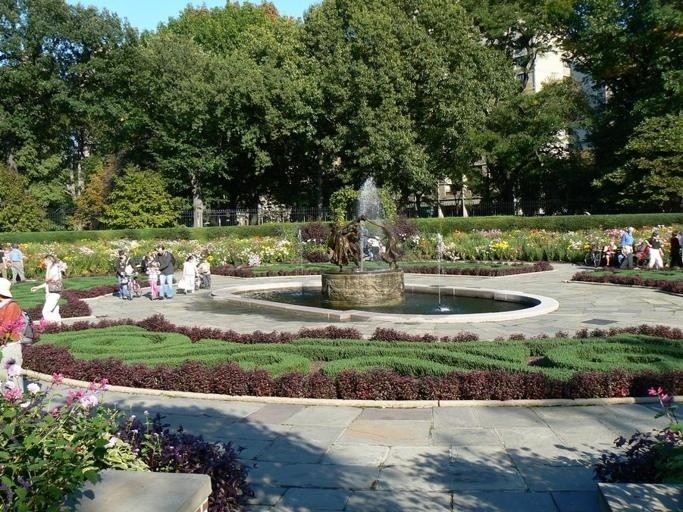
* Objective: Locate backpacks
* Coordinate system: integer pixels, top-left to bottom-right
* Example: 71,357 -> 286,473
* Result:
5,300 -> 34,344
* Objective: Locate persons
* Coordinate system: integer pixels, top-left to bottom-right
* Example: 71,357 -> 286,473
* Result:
116,246 -> 212,300
599,226 -> 682,270
0,278 -> 26,394
30,254 -> 66,323
7,243 -> 27,282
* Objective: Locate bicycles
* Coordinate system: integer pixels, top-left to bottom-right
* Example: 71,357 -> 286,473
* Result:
127,275 -> 141,300
583,244 -> 600,267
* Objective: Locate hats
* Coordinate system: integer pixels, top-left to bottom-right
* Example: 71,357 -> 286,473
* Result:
0,278 -> 13,298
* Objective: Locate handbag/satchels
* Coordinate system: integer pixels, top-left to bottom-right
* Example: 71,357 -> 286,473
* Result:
49,279 -> 62,293
125,264 -> 134,274
